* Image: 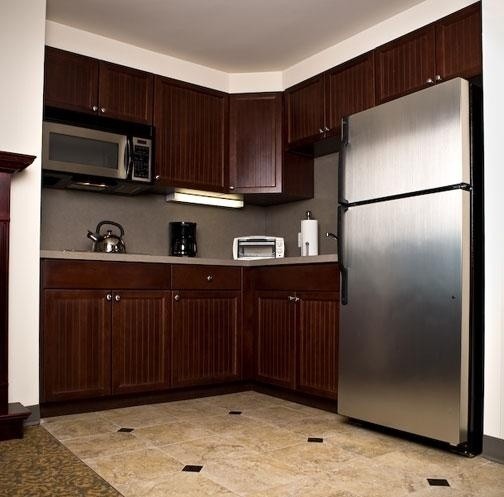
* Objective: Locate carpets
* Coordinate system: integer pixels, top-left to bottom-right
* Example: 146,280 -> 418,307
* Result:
0,421 -> 126,497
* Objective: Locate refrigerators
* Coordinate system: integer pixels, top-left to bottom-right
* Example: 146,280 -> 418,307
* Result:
337,76 -> 469,446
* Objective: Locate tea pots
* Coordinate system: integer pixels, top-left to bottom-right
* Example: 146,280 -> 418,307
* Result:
86,220 -> 126,253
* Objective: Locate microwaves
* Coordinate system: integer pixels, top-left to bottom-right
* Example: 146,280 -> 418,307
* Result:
43,105 -> 155,184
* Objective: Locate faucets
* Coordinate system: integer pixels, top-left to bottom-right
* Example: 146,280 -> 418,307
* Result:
326,232 -> 337,241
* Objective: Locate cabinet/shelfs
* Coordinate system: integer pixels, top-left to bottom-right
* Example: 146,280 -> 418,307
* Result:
40,255 -> 171,402
286,51 -> 376,158
248,264 -> 339,401
44,45 -> 154,123
171,264 -> 247,390
153,74 -> 314,206
373,1 -> 481,104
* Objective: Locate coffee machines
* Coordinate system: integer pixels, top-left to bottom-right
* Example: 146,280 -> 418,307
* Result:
168,221 -> 196,258
